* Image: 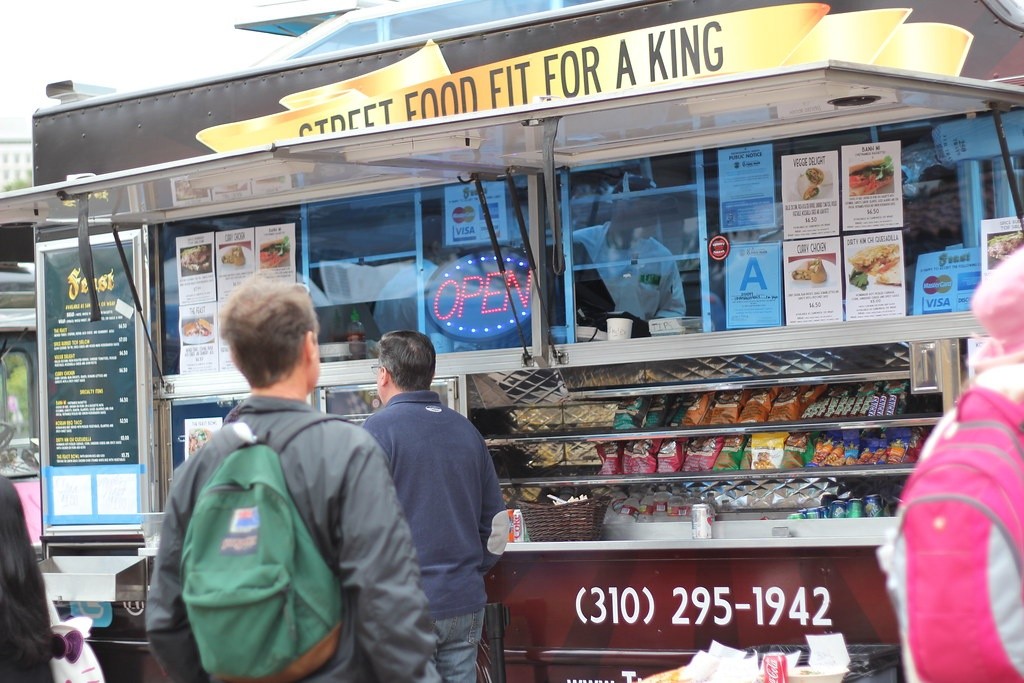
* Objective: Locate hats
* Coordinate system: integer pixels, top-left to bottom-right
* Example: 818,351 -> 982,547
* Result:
971,245 -> 1024,351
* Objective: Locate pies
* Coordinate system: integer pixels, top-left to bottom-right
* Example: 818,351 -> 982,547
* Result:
796,167 -> 832,200
792,257 -> 827,283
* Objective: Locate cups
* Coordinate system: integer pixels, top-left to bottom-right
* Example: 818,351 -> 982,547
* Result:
606,317 -> 633,341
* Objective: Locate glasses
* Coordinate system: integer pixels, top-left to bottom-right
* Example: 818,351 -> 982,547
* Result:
372,364 -> 381,374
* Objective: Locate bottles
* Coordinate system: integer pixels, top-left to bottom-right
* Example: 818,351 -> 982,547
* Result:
347,307 -> 367,359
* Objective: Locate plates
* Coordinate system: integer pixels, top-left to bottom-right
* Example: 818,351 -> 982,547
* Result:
182,325 -> 217,345
220,245 -> 253,271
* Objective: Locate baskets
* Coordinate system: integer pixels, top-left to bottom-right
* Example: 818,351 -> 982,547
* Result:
515,490 -> 611,541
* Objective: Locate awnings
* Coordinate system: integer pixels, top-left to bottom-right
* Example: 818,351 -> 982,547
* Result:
0,64 -> 1024,395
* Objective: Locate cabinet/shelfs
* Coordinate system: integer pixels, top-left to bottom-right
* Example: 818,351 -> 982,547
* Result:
466,339 -> 960,521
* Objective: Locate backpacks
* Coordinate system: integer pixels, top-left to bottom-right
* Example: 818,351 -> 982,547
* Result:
875,382 -> 1024,683
180,413 -> 347,683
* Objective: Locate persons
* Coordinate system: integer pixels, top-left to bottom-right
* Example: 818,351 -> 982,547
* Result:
886,239 -> 1024,682
0,476 -> 58,683
144,271 -> 438,681
360,328 -> 510,682
563,177 -> 687,322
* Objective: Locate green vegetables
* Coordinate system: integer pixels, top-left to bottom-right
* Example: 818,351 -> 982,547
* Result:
850,155 -> 894,181
848,269 -> 868,291
262,236 -> 291,257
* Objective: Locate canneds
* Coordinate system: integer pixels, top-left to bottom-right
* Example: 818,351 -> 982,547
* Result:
690,503 -> 712,539
787,493 -> 884,518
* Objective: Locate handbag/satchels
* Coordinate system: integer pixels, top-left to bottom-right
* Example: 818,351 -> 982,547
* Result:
45,592 -> 105,683
